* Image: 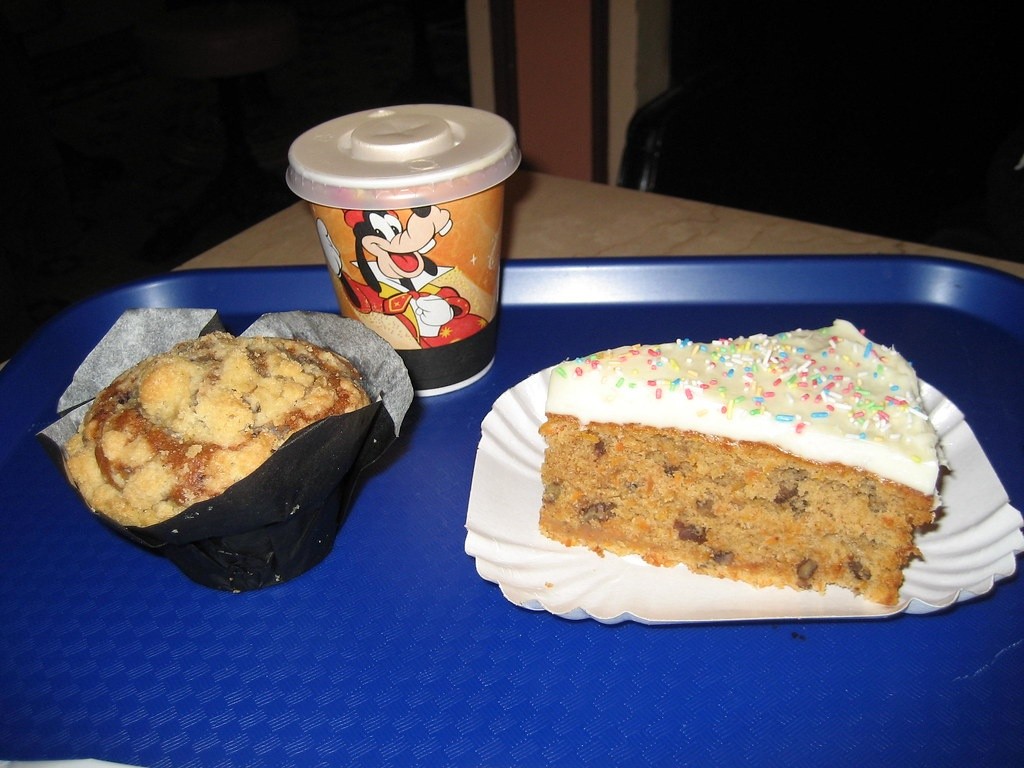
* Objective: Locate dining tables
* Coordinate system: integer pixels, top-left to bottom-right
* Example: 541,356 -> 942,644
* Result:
0,168 -> 1024,768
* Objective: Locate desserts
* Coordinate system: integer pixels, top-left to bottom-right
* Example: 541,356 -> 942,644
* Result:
62,329 -> 377,592
537,319 -> 946,608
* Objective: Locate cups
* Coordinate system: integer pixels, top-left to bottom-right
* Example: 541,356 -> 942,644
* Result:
286,100 -> 524,403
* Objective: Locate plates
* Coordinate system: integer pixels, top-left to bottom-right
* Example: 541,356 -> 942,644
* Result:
462,322 -> 1020,628
